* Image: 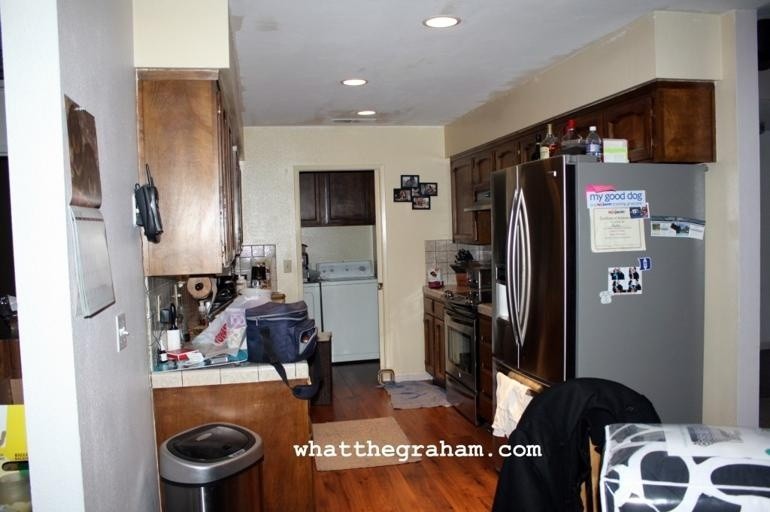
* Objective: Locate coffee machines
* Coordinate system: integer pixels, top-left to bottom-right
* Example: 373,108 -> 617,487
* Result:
251,266 -> 266,288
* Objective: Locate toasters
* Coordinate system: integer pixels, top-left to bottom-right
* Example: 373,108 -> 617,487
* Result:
467,267 -> 492,290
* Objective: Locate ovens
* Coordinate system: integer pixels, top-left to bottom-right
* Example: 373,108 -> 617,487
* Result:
442,304 -> 478,428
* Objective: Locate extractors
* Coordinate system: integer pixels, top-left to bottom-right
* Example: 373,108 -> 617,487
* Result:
463,191 -> 491,213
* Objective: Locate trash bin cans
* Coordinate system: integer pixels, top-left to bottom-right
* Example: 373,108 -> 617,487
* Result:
158,422 -> 266,512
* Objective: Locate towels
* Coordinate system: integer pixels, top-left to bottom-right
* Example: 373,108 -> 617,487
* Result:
491,371 -> 534,440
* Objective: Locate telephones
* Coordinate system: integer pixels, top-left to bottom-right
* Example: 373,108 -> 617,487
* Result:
135,164 -> 164,236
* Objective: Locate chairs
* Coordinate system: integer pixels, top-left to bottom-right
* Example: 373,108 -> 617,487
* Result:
491,378 -> 664,512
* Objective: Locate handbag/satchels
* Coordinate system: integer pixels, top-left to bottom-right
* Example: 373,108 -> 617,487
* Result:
245,301 -> 317,362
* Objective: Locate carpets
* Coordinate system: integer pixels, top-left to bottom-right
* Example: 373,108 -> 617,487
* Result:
379,376 -> 453,410
311,417 -> 422,472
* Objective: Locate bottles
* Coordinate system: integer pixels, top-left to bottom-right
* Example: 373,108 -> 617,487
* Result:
540,118 -> 602,163
158,349 -> 168,363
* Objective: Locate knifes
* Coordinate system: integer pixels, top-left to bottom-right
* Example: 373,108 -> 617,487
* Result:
454,248 -> 473,265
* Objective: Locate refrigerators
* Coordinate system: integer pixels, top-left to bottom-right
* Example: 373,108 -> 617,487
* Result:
489,153 -> 707,476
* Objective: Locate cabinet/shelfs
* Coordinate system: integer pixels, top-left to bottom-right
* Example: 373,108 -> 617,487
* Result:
298,171 -> 324,228
322,170 -> 375,227
476,312 -> 493,434
444,370 -> 478,425
474,127 -> 522,245
608,79 -> 719,165
135,67 -> 244,275
522,115 -> 552,167
419,293 -> 434,382
552,99 -> 608,157
435,300 -> 446,387
446,143 -> 474,244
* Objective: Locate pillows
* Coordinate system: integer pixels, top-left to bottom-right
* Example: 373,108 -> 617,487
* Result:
598,422 -> 770,512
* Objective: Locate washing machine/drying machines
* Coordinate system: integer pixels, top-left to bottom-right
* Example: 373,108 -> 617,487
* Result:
317,261 -> 379,367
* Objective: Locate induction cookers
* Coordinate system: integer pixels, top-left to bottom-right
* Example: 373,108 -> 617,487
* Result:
444,290 -> 493,307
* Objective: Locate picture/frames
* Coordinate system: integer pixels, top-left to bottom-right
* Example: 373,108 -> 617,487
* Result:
393,175 -> 437,208
